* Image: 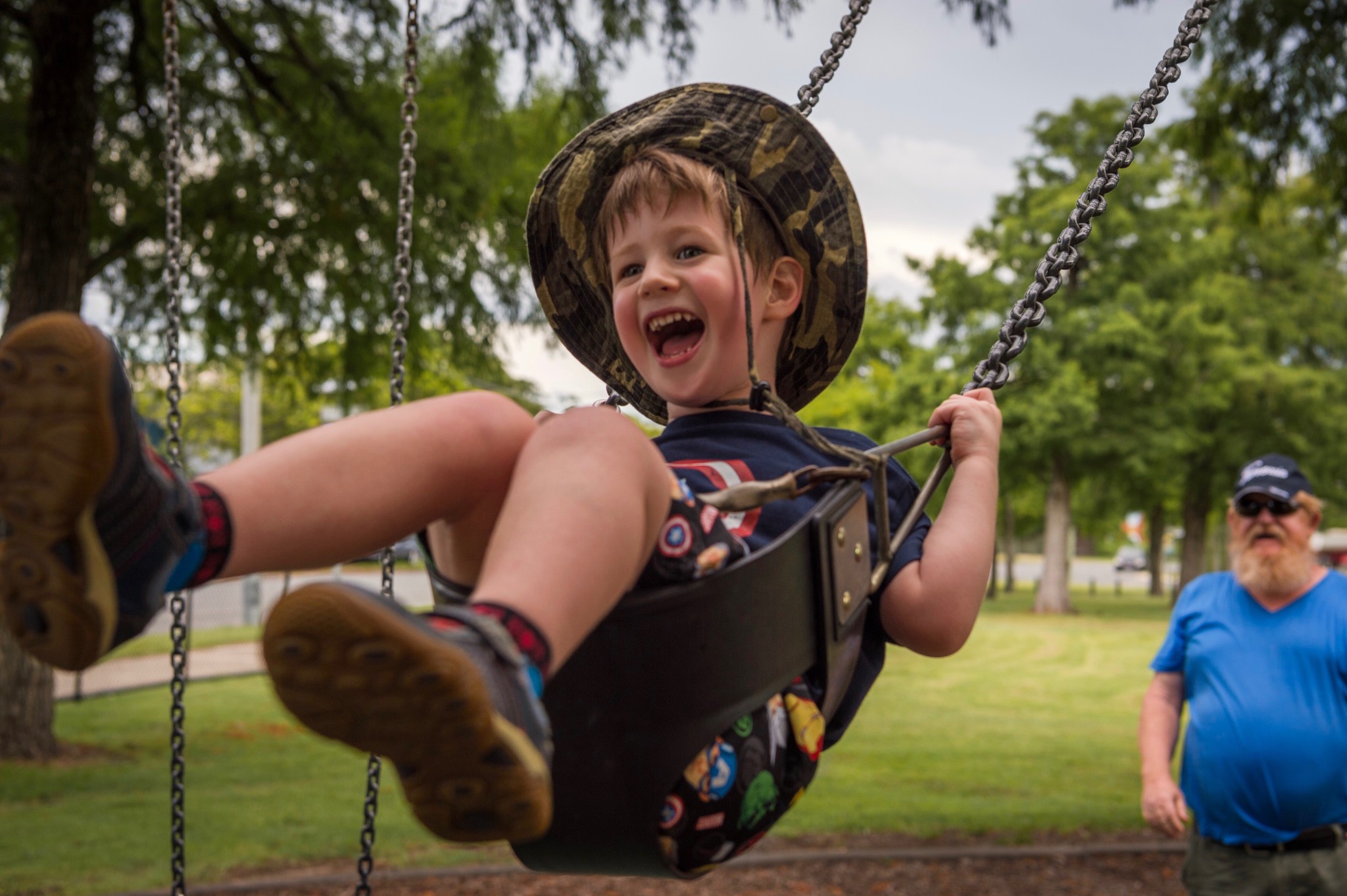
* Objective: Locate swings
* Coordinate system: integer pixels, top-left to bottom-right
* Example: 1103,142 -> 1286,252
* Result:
404,1 -> 1228,880
160,0 -> 428,896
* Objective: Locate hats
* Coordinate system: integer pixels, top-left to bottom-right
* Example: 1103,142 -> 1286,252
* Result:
527,82 -> 865,417
1234,454 -> 1310,508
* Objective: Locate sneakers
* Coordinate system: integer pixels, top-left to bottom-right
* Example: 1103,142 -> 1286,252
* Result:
262,583 -> 557,851
0,312 -> 187,672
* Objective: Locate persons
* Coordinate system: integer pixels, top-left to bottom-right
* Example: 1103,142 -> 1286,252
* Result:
1146,445 -> 1345,893
4,82 -> 1005,875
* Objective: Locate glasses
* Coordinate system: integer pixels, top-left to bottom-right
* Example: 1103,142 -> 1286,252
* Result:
1233,500 -> 1304,520
